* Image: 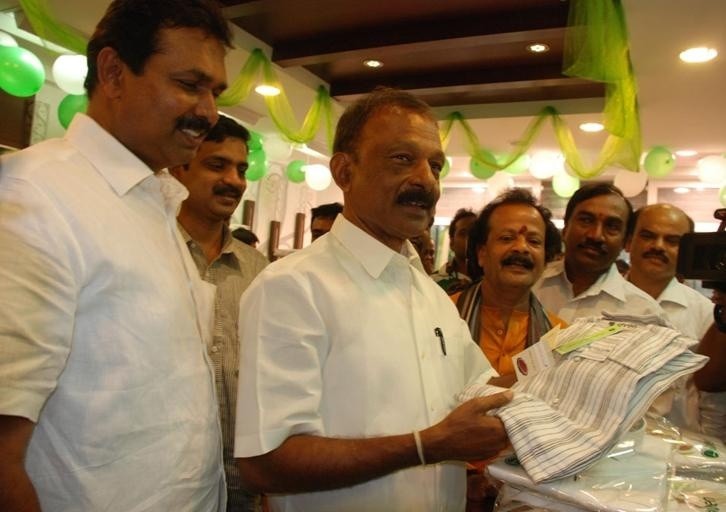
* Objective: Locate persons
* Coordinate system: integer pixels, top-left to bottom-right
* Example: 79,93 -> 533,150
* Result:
169,111 -> 725,510
228,78 -> 515,512
0,1 -> 234,512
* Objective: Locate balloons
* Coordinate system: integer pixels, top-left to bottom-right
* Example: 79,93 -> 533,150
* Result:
0,30 -> 18,47
57,94 -> 88,129
0,44 -> 45,97
51,52 -> 89,96
